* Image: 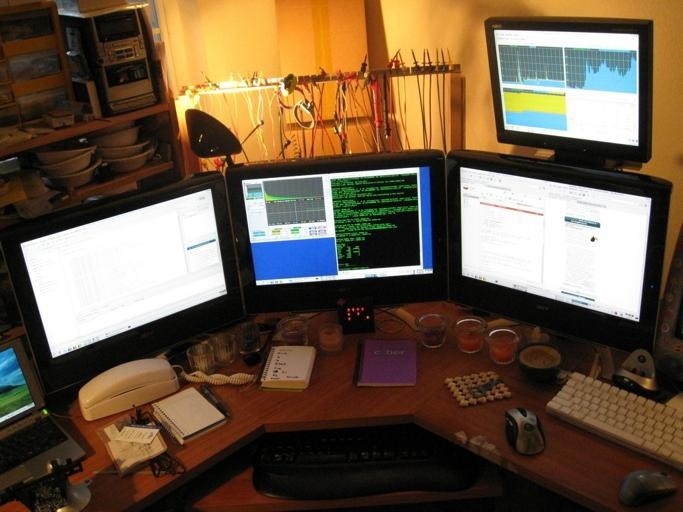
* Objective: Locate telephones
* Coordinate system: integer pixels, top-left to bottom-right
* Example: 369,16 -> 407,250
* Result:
78,358 -> 180,421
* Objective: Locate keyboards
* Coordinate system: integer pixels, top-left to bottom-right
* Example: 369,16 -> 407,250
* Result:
545,371 -> 683,471
248,424 -> 483,500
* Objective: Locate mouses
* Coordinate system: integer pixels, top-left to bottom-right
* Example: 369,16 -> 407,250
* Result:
505,408 -> 545,454
619,467 -> 675,506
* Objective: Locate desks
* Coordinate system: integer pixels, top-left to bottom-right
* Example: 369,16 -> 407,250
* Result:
0,298 -> 682,512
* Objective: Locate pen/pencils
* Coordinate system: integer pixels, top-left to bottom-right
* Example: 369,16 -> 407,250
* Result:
200,384 -> 232,420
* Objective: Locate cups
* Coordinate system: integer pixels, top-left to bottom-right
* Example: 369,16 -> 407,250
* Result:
415,311 -> 564,381
185,316 -> 343,374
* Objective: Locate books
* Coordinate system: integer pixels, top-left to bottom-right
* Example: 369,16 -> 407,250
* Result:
354,337 -> 418,388
259,345 -> 317,392
153,387 -> 227,448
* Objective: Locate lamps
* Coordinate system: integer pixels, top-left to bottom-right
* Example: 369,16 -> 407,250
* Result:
185,108 -> 242,167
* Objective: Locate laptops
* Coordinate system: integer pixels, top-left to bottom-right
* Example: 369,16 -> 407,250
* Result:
0,338 -> 87,503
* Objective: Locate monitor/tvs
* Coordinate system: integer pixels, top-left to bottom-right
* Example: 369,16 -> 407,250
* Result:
226,150 -> 449,343
0,171 -> 246,402
446,148 -> 673,378
484,15 -> 653,170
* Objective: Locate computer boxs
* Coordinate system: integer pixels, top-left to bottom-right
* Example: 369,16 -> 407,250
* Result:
56,8 -> 157,115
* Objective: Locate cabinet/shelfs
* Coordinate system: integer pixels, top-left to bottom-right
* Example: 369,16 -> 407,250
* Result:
0,0 -> 186,347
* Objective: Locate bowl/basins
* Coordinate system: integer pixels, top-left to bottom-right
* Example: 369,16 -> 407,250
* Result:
34,127 -> 154,188
